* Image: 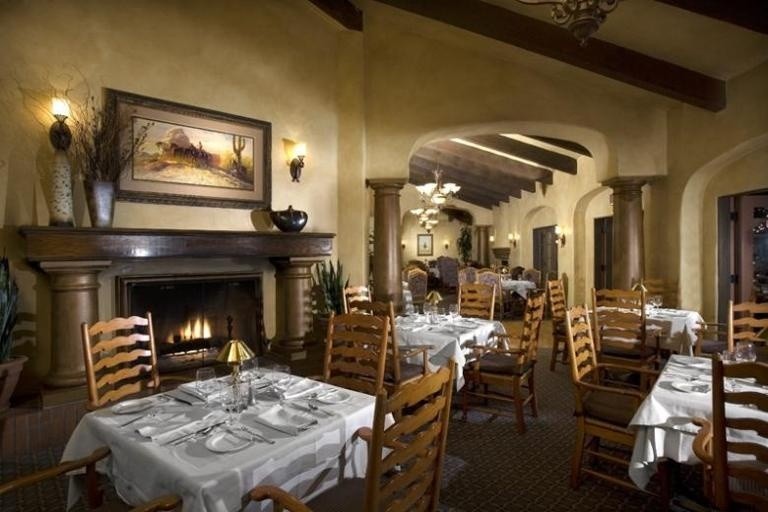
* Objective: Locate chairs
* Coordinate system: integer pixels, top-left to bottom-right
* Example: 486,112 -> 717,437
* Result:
0,445 -> 182,512
80,312 -> 160,408
250,359 -> 458,512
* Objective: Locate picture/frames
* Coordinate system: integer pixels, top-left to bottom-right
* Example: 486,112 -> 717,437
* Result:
102,87 -> 272,210
417,234 -> 433,256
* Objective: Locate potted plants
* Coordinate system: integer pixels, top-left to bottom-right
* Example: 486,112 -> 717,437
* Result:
0,257 -> 29,412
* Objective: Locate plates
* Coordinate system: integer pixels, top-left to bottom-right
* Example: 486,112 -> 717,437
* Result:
112,371 -> 352,452
670,381 -> 711,394
673,356 -> 710,367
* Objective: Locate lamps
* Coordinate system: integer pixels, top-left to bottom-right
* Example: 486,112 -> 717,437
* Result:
290,142 -> 307,183
554,226 -> 565,245
217,339 -> 256,400
49,97 -> 72,151
508,232 -> 521,248
415,151 -> 461,204
517,0 -> 621,49
409,200 -> 440,233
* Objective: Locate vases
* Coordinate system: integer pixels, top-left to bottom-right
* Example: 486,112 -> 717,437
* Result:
48,152 -> 74,227
83,179 -> 116,228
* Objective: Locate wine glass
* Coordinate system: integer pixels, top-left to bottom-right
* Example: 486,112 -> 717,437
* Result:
196,357 -> 290,425
722,342 -> 756,390
647,295 -> 663,316
398,301 -> 460,330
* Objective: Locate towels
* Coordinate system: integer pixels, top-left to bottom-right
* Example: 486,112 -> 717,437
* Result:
274,377 -> 321,399
178,380 -> 227,401
134,408 -> 225,445
253,403 -> 318,436
